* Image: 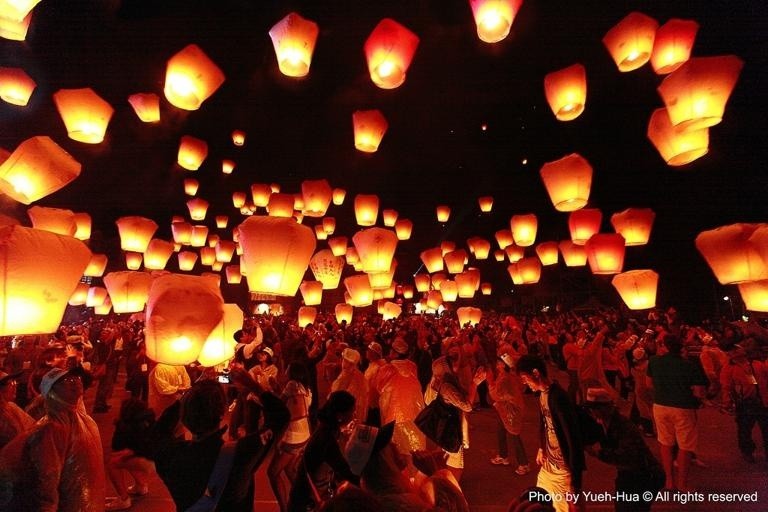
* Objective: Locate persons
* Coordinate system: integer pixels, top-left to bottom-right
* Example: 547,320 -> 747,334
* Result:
0,305 -> 767,510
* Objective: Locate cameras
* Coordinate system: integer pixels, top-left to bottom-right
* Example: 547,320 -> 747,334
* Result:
215,374 -> 230,384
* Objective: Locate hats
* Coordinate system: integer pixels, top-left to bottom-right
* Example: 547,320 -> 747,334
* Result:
575,388 -> 611,408
346,419 -> 395,476
40,367 -> 83,397
0,368 -> 23,383
497,353 -> 512,368
262,347 -> 273,358
342,340 -> 409,362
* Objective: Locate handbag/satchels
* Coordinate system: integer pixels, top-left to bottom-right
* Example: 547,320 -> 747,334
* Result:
414,399 -> 462,453
